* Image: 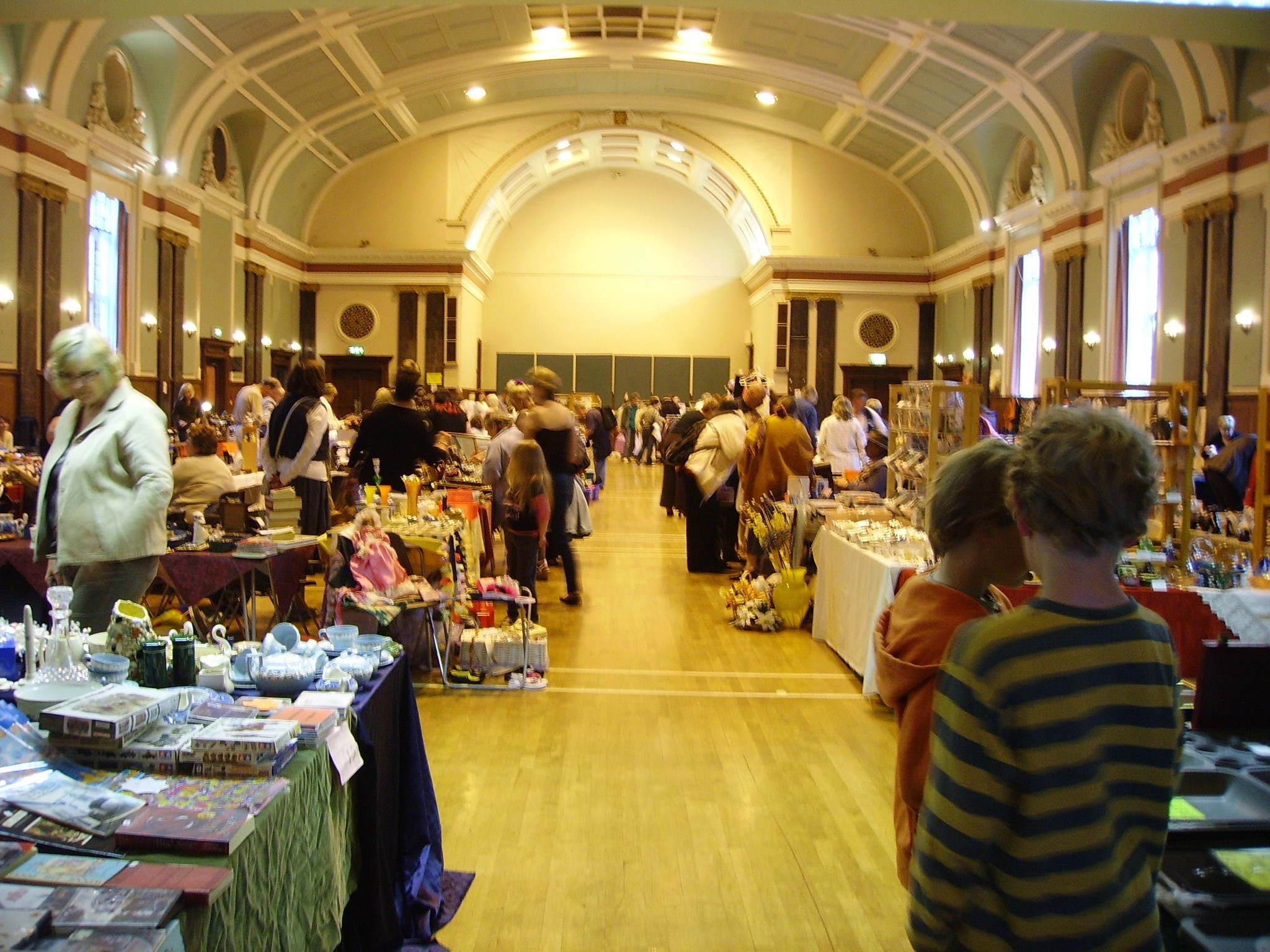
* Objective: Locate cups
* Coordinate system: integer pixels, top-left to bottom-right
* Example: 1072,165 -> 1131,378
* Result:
29,527 -> 36,549
816,478 -> 829,499
39,633 -> 83,668
417,489 -> 447,516
1137,549 -> 1166,575
318,625 -> 393,652
83,631 -> 108,654
0,514 -> 16,541
83,653 -> 130,685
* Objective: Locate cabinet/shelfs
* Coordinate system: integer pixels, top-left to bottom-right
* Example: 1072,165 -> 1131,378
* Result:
884,379 -> 984,535
1042,376 -> 1197,574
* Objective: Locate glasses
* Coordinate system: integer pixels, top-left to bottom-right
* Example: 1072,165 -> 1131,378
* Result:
57,370 -> 99,383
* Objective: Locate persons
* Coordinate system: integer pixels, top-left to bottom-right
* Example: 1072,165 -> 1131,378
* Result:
31,323 -> 175,635
870,439 -> 1030,890
258,360 -> 333,536
231,376 -> 281,425
171,382 -> 204,442
261,386 -> 287,424
319,382 -> 352,433
348,358 -> 887,683
0,414 -> 14,450
905,399 -> 1186,952
1198,415 -> 1257,515
168,423 -> 237,531
39,397 -> 74,461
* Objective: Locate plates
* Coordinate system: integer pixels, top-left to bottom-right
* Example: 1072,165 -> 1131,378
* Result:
161,686 -> 234,708
318,640 -> 394,667
229,670 -> 323,689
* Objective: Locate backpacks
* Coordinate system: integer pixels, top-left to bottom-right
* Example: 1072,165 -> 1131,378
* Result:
587,406 -> 617,430
666,410 -> 736,467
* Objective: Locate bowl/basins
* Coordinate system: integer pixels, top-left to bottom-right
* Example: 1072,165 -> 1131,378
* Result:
1139,568 -> 1197,591
1117,564 -> 1138,586
14,680 -> 139,722
784,493 -> 804,506
206,536 -> 244,553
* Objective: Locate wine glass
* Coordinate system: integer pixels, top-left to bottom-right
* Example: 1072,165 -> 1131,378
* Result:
364,486 -> 391,506
401,474 -> 423,521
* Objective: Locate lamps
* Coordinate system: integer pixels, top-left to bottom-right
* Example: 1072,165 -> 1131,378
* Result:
463,85 -> 486,102
753,82 -> 778,104
933,310 -> 1255,365
0,287 -> 303,354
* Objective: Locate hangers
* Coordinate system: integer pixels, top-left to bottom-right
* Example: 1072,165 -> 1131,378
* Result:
738,365 -> 775,387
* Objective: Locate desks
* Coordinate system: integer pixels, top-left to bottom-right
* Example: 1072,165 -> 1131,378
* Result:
328,499 -> 495,590
42,650 -> 474,952
1,536 -> 319,641
893,567 -> 1270,681
812,526 -> 918,697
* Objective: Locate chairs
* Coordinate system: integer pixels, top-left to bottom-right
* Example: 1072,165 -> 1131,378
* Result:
322,531 -> 449,688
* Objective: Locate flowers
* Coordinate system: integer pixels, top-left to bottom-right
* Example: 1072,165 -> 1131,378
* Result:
720,488 -> 794,633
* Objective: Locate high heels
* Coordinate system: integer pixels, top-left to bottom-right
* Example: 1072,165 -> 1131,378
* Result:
666,506 -> 674,517
678,509 -> 687,517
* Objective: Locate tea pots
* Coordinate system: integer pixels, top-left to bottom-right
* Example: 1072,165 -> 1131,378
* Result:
246,646 -> 322,699
317,648 -> 379,698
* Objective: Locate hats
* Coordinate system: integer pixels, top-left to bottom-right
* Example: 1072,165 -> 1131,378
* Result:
530,365 -> 561,391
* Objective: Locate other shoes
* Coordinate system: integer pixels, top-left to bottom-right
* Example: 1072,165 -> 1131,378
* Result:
560,593 -> 582,607
620,454 -> 665,466
729,570 -> 757,581
536,559 -> 550,580
592,483 -> 603,490
722,549 -> 747,562
718,566 -> 739,575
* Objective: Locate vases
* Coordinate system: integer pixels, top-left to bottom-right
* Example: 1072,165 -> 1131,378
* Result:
401,474 -> 419,519
771,566 -> 812,628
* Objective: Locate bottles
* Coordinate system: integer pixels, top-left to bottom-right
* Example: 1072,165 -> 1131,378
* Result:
33,586 -> 88,682
1230,548 -> 1270,590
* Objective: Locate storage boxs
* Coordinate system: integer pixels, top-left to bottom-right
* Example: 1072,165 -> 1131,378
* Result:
508,622 -> 547,639
218,501 -> 255,539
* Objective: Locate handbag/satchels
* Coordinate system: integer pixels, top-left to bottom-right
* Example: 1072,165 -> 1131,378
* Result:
261,474 -> 270,494
615,432 -> 625,451
